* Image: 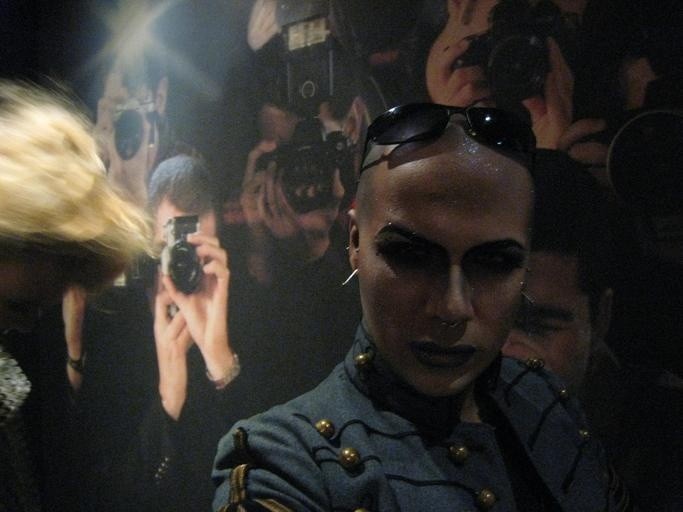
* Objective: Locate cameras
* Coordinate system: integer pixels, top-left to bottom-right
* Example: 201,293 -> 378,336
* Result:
114,87 -> 156,159
447,1 -> 563,103
164,216 -> 203,293
257,128 -> 352,211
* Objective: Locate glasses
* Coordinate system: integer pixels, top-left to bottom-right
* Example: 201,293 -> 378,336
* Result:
360,105 -> 538,187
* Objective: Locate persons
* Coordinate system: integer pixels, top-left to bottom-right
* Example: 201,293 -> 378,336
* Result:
0,0 -> 683,512
